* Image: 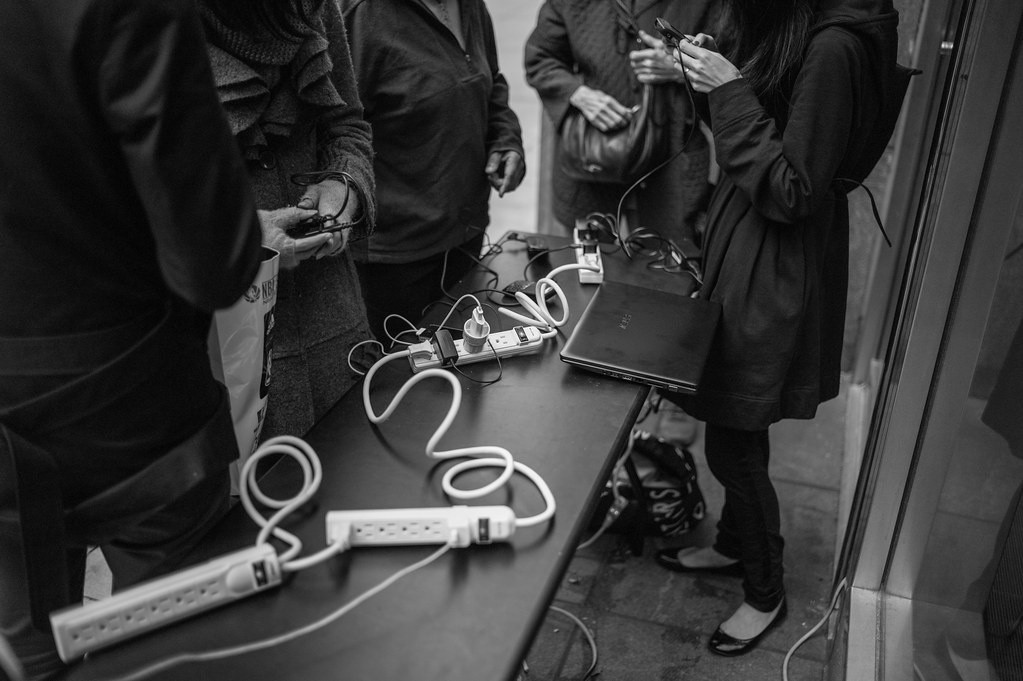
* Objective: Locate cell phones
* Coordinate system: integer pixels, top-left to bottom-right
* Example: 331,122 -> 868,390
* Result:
503,279 -> 560,302
527,235 -> 548,254
654,17 -> 692,44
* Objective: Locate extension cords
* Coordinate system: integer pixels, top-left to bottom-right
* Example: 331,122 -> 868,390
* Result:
572,228 -> 604,284
407,326 -> 544,374
48,543 -> 282,662
324,505 -> 513,545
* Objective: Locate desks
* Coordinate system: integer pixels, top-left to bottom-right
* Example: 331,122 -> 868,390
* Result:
63,228 -> 699,681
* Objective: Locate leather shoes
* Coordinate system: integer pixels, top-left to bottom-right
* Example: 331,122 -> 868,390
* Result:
655,544 -> 757,577
707,596 -> 789,657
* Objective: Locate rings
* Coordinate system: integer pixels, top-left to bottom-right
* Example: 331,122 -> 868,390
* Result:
684,68 -> 691,78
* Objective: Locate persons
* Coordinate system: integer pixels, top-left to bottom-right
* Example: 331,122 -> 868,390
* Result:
654,0 -> 923,656
1,1 -> 261,620
335,0 -> 527,353
524,0 -> 716,477
194,0 -> 383,481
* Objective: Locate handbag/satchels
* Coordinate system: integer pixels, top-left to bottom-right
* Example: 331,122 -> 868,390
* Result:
206,242 -> 281,494
560,76 -> 664,184
585,429 -> 708,541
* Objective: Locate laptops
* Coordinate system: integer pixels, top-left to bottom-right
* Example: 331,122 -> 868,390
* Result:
561,280 -> 720,395
